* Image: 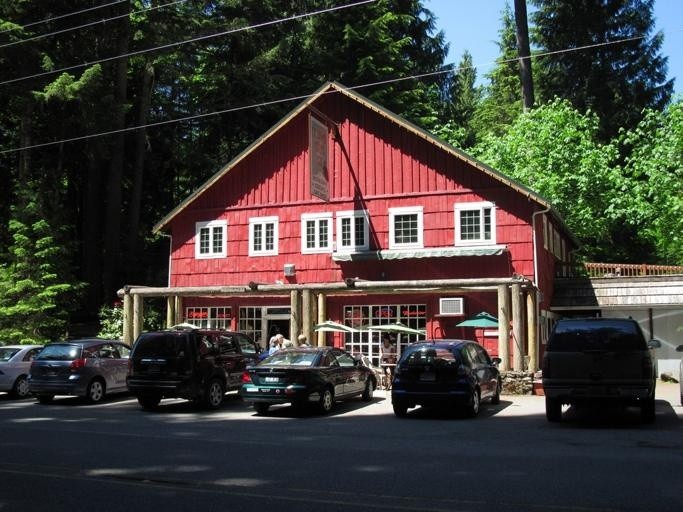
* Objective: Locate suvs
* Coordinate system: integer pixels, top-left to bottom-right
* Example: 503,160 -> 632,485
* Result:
124,326 -> 270,412
541,314 -> 661,425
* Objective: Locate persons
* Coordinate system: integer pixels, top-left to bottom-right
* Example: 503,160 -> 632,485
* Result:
296,333 -> 313,356
276,333 -> 302,360
268,335 -> 285,364
380,336 -> 395,388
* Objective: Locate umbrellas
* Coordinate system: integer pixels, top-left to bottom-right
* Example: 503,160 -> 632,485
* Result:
298,317 -> 361,346
354,321 -> 427,354
160,320 -> 203,331
456,311 -> 512,349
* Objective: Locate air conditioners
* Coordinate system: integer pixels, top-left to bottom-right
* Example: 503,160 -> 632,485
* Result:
438,297 -> 465,314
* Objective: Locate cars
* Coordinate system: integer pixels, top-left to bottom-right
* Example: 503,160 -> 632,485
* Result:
391,336 -> 503,418
0,344 -> 44,399
26,340 -> 137,403
675,343 -> 683,406
237,344 -> 379,417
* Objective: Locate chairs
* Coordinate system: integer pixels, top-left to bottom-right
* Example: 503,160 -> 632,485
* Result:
360,355 -> 385,390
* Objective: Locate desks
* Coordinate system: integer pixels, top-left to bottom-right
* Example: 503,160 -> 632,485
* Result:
380,362 -> 396,390
